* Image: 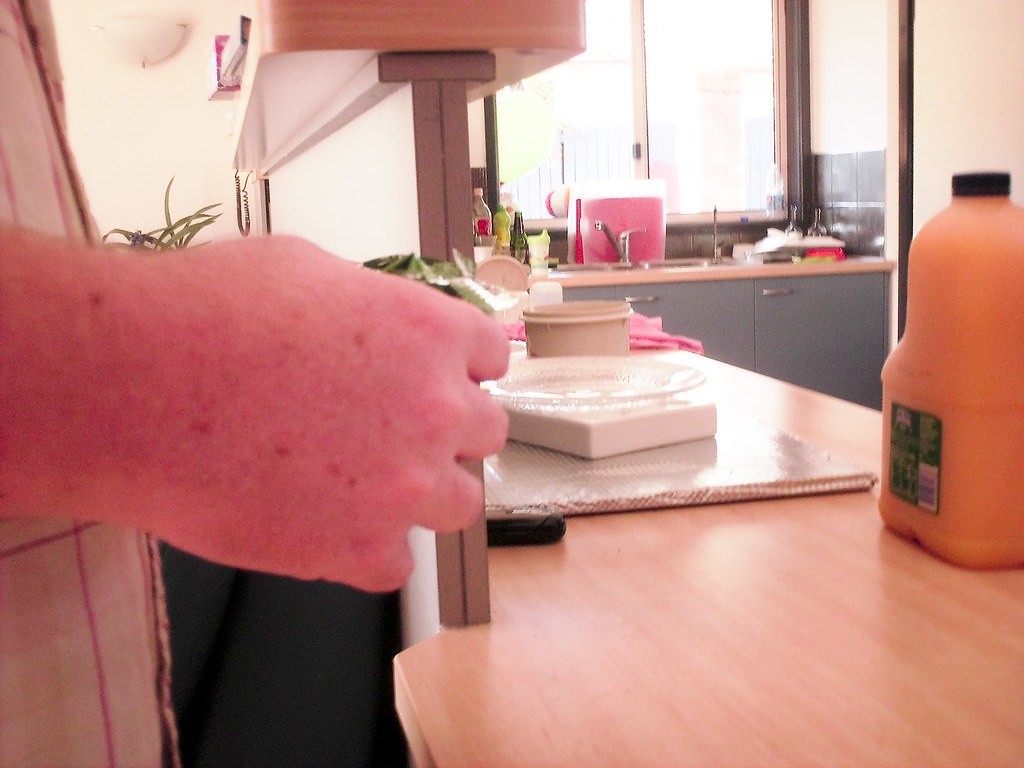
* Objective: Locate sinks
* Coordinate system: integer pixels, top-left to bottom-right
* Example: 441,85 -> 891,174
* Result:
551,252 -> 710,276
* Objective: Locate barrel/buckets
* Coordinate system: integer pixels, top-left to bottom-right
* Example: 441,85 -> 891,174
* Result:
520,298 -> 634,358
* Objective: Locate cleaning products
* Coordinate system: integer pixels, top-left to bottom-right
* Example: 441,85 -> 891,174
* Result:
470,184 -> 510,244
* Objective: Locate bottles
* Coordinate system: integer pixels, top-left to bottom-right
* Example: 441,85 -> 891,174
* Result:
508,212 -> 530,268
472,187 -> 491,249
492,206 -> 511,260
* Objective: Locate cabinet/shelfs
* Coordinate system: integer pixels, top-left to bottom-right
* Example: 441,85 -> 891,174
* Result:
563,272 -> 890,413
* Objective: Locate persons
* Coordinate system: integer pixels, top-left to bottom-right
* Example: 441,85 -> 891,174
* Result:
0,0 -> 510,768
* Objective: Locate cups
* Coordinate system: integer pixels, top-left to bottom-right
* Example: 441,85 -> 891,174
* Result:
880,168 -> 1024,572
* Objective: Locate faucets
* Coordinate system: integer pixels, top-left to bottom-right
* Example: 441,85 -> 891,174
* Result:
593,221 -> 647,262
